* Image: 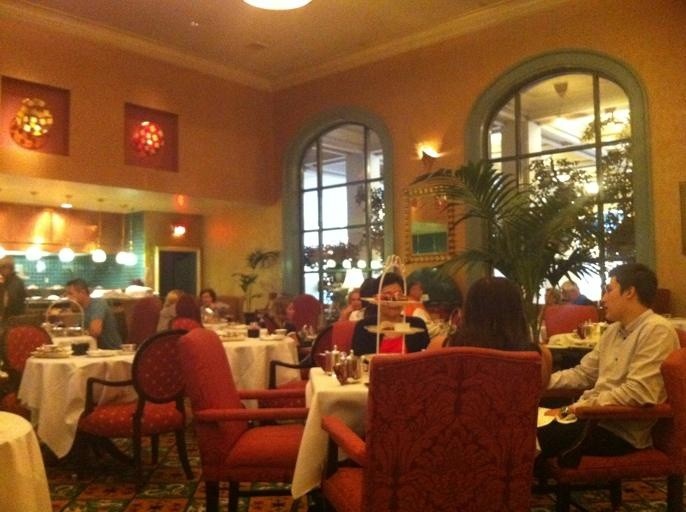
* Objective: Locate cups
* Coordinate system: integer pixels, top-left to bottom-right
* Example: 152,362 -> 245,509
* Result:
259,328 -> 268,336
121,343 -> 135,351
275,328 -> 287,336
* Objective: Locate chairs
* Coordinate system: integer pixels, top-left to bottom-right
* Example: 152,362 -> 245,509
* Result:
286,295 -> 319,337
316,347 -> 540,511
3,323 -> 51,424
179,328 -> 323,511
543,303 -> 599,342
71,330 -> 193,493
548,347 -> 684,512
258,320 -> 356,429
122,298 -> 159,346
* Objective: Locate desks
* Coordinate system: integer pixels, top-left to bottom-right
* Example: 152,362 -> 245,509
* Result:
18,347 -> 143,478
0,410 -> 52,512
547,322 -> 604,378
208,333 -> 300,425
292,364 -> 368,508
202,319 -> 288,334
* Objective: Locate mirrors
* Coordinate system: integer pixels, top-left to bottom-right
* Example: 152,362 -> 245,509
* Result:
401,184 -> 457,264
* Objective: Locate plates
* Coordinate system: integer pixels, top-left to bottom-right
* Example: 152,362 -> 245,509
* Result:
260,336 -> 283,340
363,354 -> 398,362
362,297 -> 429,306
52,326 -> 82,332
31,349 -> 73,358
85,350 -> 116,356
365,324 -> 422,336
117,350 -> 135,355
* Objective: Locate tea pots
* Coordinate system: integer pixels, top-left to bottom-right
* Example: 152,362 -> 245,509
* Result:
315,345 -> 346,375
333,350 -> 369,384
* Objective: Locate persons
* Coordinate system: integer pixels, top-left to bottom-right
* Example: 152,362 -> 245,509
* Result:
562,281 -> 592,307
63,278 -> 122,350
198,290 -> 239,326
535,262 -> 681,479
334,269 -> 440,357
0,257 -> 26,332
168,295 -> 204,334
538,287 -> 561,332
441,277 -> 540,358
266,292 -> 301,351
155,288 -> 180,335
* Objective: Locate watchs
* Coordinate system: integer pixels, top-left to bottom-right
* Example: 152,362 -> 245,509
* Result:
560,405 -> 568,417
